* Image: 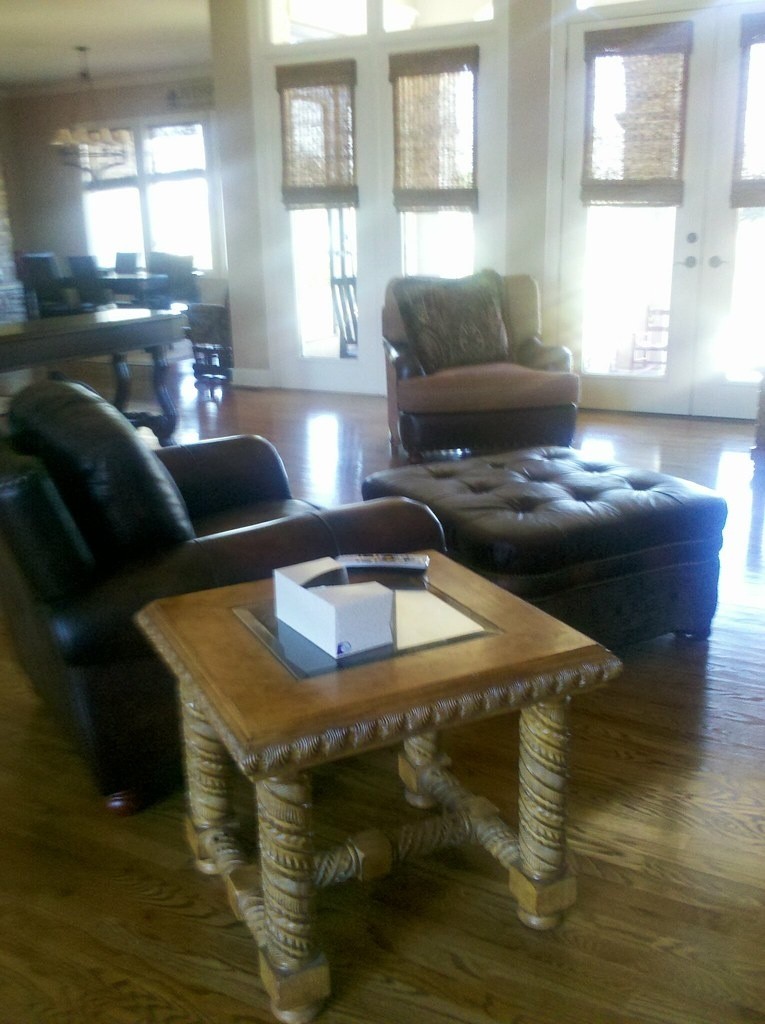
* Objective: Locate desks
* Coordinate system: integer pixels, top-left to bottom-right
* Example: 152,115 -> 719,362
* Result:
0,308 -> 187,448
132,548 -> 625,1023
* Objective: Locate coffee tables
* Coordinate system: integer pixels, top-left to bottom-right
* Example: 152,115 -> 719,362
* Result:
362,445 -> 729,653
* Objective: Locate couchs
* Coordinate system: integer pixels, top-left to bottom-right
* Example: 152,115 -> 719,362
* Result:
1,378 -> 451,814
381,275 -> 580,461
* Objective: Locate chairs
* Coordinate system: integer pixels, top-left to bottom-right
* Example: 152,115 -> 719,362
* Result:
330,275 -> 358,359
629,307 -> 672,373
19,250 -> 203,353
187,304 -> 234,380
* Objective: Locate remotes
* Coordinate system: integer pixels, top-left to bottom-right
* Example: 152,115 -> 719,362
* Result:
335,553 -> 430,577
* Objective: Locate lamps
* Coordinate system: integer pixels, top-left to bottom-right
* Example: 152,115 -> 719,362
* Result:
44,46 -> 129,187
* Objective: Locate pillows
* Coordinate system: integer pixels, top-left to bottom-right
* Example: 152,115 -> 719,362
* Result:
392,269 -> 515,376
